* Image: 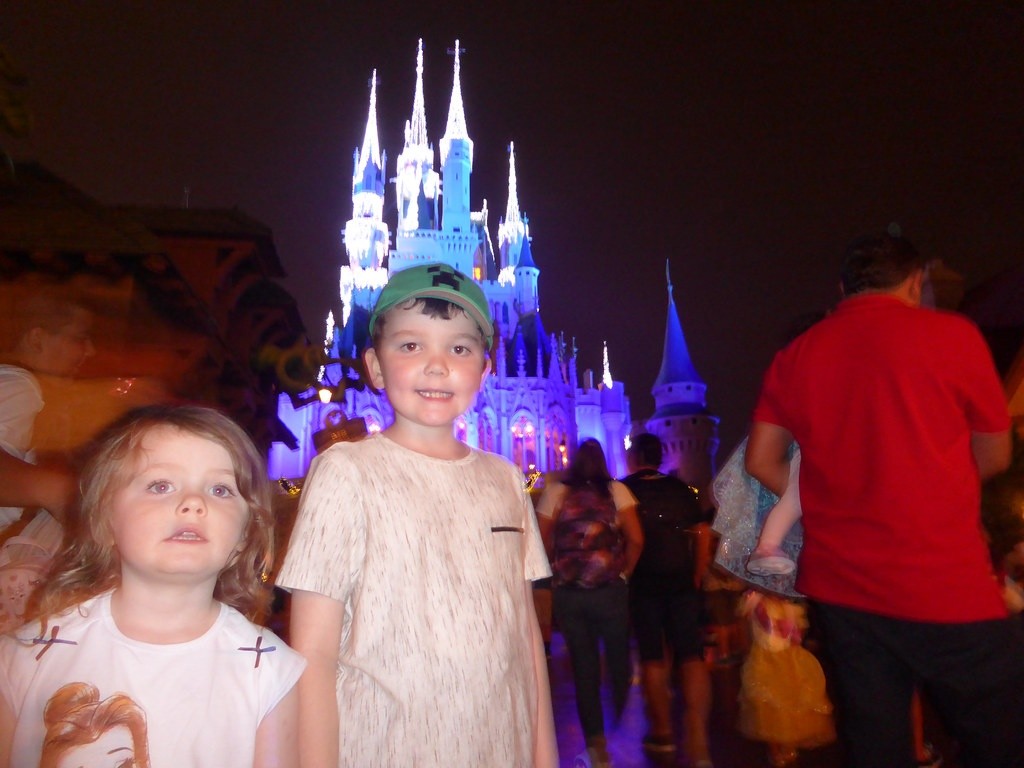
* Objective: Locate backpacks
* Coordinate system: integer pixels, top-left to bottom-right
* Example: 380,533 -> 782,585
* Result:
551,476 -> 626,588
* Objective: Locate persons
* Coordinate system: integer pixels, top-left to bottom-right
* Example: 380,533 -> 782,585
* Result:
710,429 -> 806,597
736,581 -> 837,768
618,432 -> 713,767
276,263 -> 561,768
744,234 -> 1024,767
534,437 -> 644,768
0,291 -> 179,542
0,403 -> 307,768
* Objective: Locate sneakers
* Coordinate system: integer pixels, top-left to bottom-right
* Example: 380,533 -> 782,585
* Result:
641,733 -> 678,752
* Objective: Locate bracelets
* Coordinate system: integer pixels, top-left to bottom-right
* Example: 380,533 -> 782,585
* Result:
619,571 -> 627,584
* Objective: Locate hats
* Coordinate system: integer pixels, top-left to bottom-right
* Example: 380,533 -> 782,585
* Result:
370,261 -> 494,353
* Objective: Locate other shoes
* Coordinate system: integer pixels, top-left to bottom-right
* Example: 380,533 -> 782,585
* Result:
746,550 -> 798,577
